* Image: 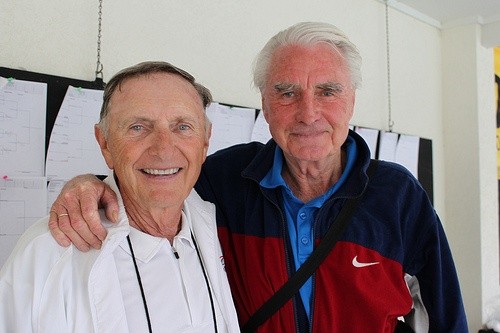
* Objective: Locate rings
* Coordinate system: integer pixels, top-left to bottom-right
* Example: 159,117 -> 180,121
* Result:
57,214 -> 68,218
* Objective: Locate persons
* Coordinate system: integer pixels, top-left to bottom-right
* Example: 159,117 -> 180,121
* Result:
48,21 -> 468,333
1,61 -> 241,333
